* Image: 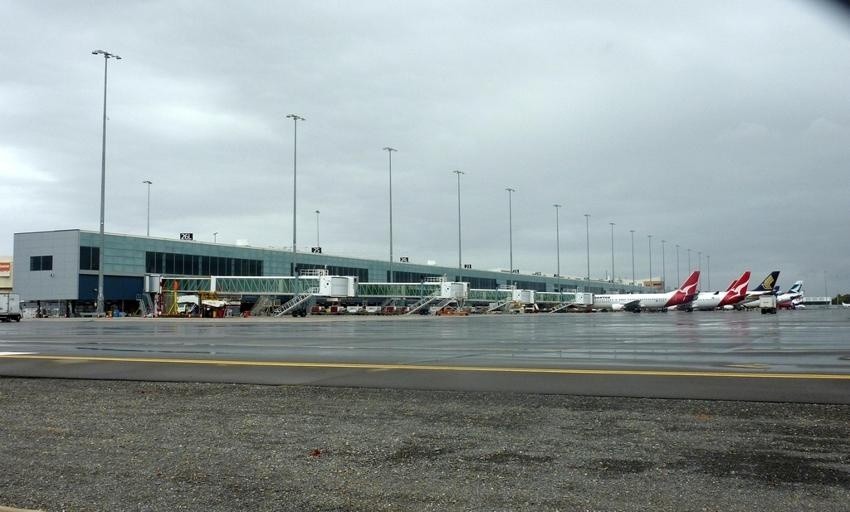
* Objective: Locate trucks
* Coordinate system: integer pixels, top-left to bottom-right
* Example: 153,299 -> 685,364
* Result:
0,292 -> 23,323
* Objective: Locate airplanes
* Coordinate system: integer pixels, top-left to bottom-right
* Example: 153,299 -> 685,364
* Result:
562,268 -> 808,314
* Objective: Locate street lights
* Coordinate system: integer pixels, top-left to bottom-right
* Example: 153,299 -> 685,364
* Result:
610,223 -> 616,294
213,231 -> 218,242
453,170 -> 465,282
505,188 -> 515,287
383,146 -> 398,282
686,249 -> 691,276
707,255 -> 711,292
553,204 -> 563,291
675,244 -> 681,289
144,179 -> 152,236
630,230 -> 636,293
286,113 -> 306,296
697,252 -> 702,291
661,240 -> 667,291
584,214 -> 591,292
315,210 -> 320,252
647,234 -> 653,292
92,50 -> 120,316
